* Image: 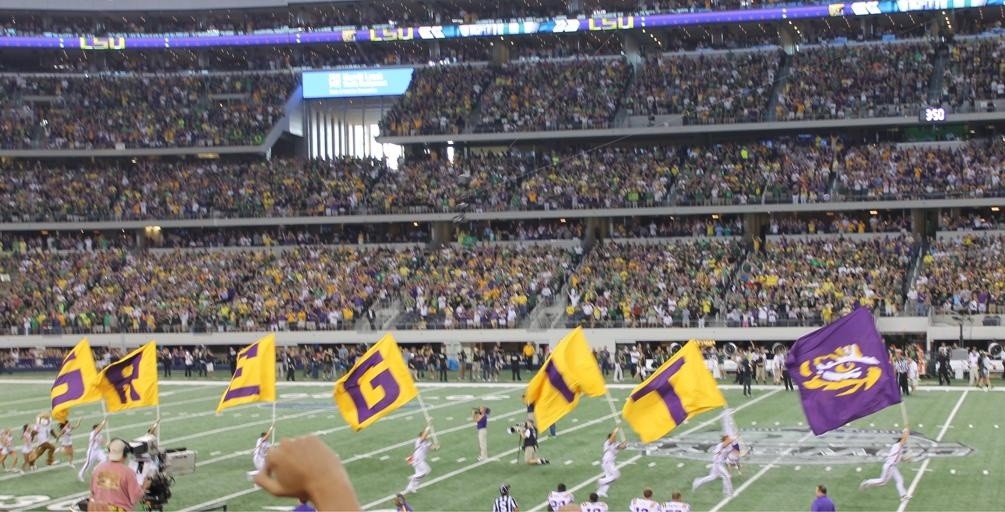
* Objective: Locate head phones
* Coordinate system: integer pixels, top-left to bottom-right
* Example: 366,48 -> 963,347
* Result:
107,437 -> 132,456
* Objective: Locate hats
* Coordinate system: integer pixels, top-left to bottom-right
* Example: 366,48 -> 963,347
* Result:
108,439 -> 125,461
500,484 -> 511,494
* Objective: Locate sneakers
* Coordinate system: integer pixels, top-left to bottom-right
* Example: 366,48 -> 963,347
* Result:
1,460 -> 85,482
598,478 -> 610,498
537,457 -> 551,465
692,478 -> 701,493
405,485 -> 417,494
